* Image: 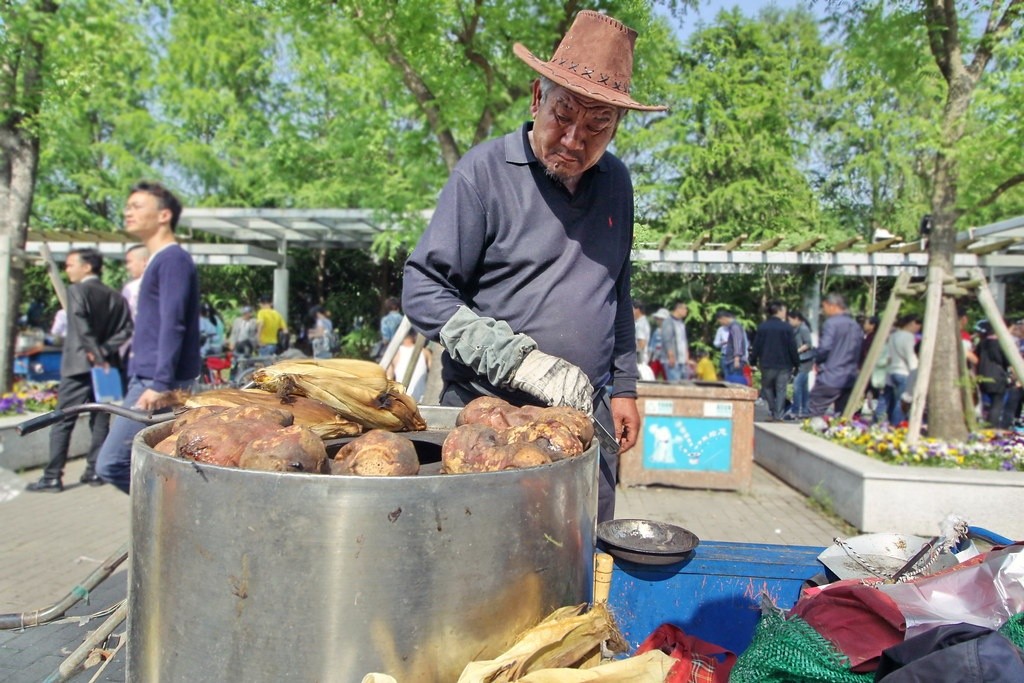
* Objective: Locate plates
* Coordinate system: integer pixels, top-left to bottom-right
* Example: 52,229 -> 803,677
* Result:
597,518 -> 700,564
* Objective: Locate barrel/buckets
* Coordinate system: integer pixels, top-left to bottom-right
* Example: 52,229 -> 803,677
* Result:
125,407 -> 598,683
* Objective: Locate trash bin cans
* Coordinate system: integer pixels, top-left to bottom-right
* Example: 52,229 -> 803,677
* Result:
618,379 -> 759,493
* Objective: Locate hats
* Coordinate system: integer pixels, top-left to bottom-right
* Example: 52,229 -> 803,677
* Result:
513,10 -> 670,111
653,308 -> 669,319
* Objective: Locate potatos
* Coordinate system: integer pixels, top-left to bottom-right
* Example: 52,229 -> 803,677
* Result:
152,393 -> 595,477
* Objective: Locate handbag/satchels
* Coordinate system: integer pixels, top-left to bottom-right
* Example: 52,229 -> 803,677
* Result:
799,351 -> 814,362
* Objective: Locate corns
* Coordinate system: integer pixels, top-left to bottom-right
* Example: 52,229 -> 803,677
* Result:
169,359 -> 428,438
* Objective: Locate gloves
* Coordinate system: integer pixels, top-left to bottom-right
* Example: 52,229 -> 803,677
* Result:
510,349 -> 594,424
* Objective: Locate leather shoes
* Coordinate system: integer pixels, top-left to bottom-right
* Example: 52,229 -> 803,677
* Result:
26,478 -> 62,493
80,472 -> 101,486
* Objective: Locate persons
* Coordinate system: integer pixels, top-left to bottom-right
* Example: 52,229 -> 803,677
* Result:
196,286 -> 439,406
25,245 -> 136,495
401,9 -> 671,537
51,302 -> 69,346
95,178 -> 200,497
25,281 -> 49,324
623,284 -> 1024,445
117,245 -> 161,327
